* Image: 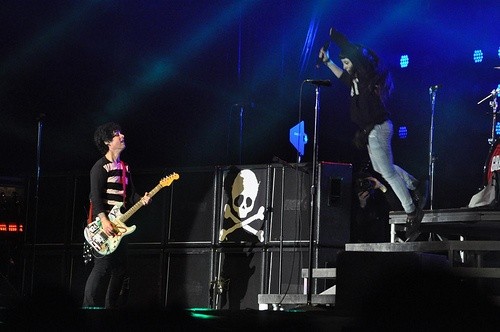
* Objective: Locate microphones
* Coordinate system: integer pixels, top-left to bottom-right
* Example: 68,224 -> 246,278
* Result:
306,79 -> 331,87
430,84 -> 445,92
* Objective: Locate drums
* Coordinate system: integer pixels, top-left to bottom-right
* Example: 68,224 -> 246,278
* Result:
483,135 -> 500,206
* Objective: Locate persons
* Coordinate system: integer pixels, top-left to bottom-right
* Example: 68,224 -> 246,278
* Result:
84,122 -> 153,307
357,177 -> 402,243
318,28 -> 427,239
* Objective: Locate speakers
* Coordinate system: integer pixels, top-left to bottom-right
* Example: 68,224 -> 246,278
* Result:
335,249 -> 449,316
0,160 -> 353,312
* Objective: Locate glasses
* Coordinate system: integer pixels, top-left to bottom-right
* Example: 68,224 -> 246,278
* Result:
110,131 -> 120,141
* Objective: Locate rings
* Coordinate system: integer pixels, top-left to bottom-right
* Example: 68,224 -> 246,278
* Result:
112,230 -> 115,233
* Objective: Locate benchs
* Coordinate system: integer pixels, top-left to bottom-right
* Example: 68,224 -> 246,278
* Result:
388,207 -> 500,278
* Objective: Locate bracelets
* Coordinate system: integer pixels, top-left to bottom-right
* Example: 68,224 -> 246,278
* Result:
325,58 -> 330,64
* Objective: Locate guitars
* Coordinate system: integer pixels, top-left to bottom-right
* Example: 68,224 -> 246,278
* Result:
83,171 -> 180,256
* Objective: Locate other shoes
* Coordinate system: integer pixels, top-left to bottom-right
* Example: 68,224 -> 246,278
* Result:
413,183 -> 427,202
405,209 -> 424,237
